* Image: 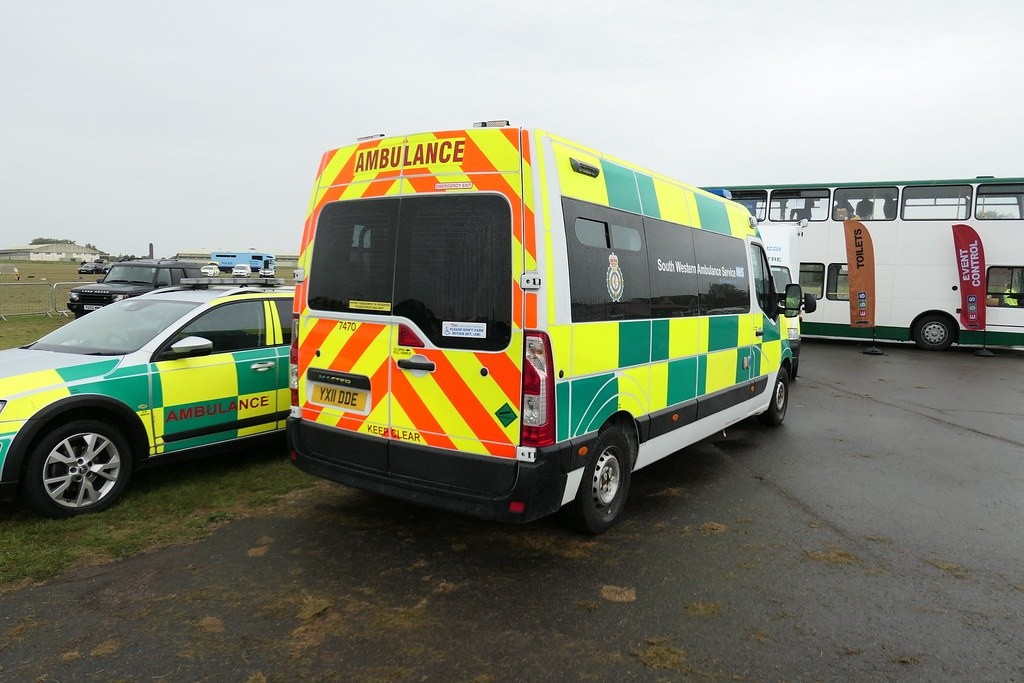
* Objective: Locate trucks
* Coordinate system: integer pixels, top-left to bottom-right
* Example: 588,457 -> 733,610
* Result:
757,218 -> 809,380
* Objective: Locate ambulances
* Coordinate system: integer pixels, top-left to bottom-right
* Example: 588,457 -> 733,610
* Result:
284,120 -> 803,536
0,279 -> 296,520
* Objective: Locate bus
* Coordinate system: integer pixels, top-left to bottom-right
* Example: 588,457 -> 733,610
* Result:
686,176 -> 1024,351
211,251 -> 277,271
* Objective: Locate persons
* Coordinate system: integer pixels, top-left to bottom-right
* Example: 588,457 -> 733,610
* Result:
883,197 -> 907,219
834,199 -> 854,218
856,198 -> 874,220
790,199 -> 815,220
1003,282 -> 1018,305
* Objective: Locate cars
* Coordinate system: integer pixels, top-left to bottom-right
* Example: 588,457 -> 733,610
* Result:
201,265 -> 220,277
78,262 -> 119,275
258,266 -> 276,277
231,264 -> 252,278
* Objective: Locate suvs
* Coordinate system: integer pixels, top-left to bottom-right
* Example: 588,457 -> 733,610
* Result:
67,258 -> 205,319
